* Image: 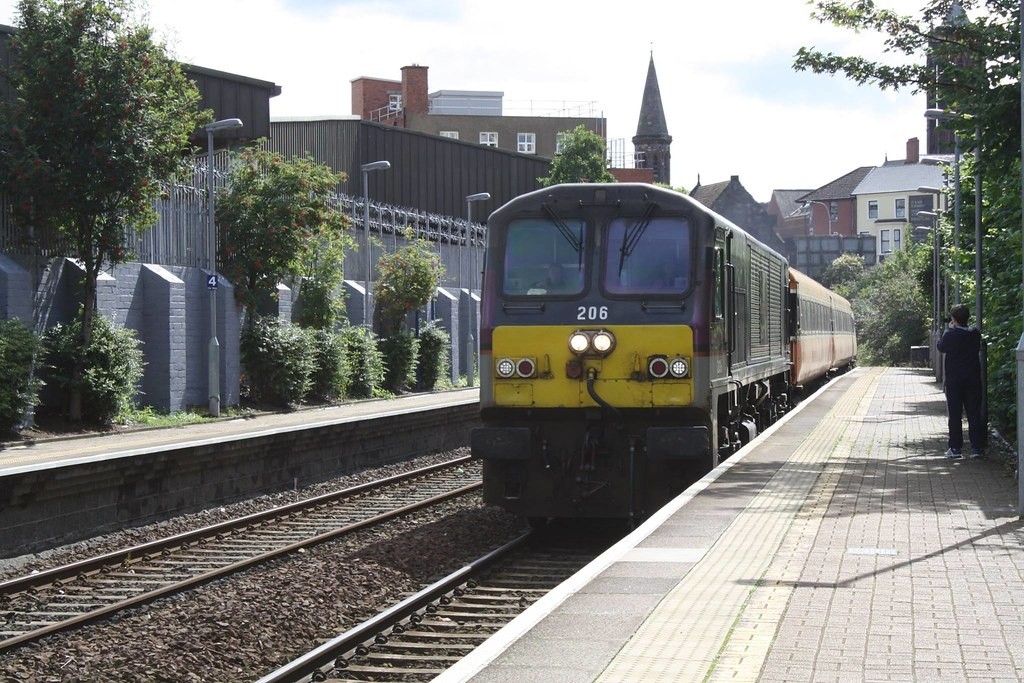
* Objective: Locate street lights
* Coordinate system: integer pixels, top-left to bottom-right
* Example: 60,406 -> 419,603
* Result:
924,109 -> 988,446
915,211 -> 938,375
465,193 -> 490,389
915,187 -> 942,383
920,157 -> 951,391
796,200 -> 832,234
360,161 -> 391,323
205,119 -> 244,414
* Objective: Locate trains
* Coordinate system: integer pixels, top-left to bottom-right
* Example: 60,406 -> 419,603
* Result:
471,183 -> 858,539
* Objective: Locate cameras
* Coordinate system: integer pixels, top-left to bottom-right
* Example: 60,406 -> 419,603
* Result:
944,318 -> 952,323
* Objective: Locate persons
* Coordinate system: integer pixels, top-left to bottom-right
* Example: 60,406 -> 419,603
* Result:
937,304 -> 984,458
529,263 -> 566,290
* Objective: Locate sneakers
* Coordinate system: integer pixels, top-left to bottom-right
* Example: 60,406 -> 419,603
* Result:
945,448 -> 962,458
970,448 -> 982,458
